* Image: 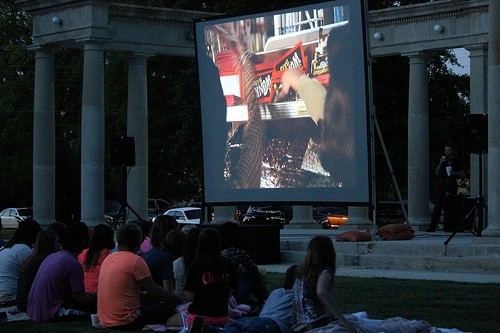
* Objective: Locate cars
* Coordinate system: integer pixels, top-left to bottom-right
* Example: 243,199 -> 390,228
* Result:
235,204 -> 348,229
0,208 -> 33,231
152,206 -> 214,225
148,198 -> 172,218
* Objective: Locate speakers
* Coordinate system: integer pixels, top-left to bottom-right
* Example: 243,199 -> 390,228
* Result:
463,113 -> 487,155
230,224 -> 280,266
110,136 -> 137,167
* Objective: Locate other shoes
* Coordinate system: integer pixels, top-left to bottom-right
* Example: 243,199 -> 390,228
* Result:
425,227 -> 436,232
189,316 -> 205,333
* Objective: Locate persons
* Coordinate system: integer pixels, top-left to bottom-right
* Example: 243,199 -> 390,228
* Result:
281,23 -> 355,188
425,143 -> 465,232
199,23 -> 263,189
291,236 -> 356,333
257,265 -> 303,333
0,215 -> 269,331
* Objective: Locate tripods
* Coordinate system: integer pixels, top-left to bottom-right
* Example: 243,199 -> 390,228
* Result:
444,154 -> 488,247
110,166 -> 144,227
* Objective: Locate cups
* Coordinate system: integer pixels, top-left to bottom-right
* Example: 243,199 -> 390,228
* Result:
446,167 -> 452,176
91,315 -> 99,326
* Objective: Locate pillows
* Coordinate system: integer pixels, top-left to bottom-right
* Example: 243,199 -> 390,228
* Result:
378,223 -> 414,240
339,231 -> 371,241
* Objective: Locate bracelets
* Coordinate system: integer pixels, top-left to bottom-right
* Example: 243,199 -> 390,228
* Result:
334,311 -> 346,321
239,50 -> 252,66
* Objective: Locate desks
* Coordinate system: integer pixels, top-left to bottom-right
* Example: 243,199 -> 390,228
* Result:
129,223 -> 284,265
